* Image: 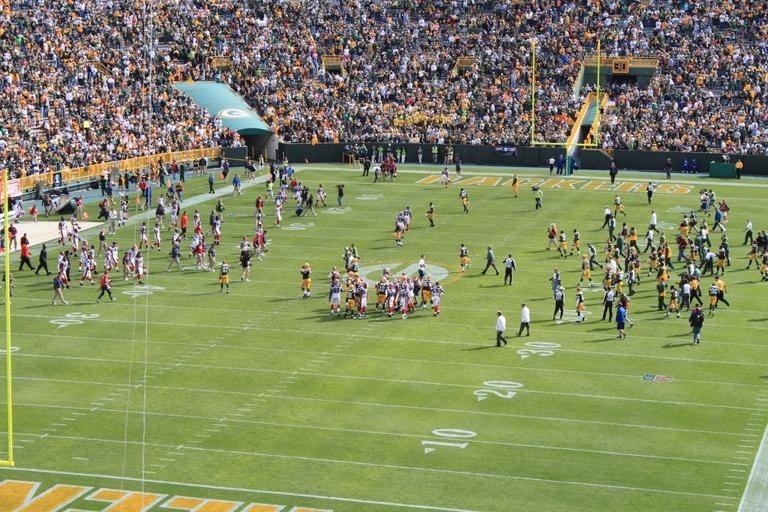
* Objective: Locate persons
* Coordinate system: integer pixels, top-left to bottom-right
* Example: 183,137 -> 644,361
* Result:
2,3 -> 767,350
